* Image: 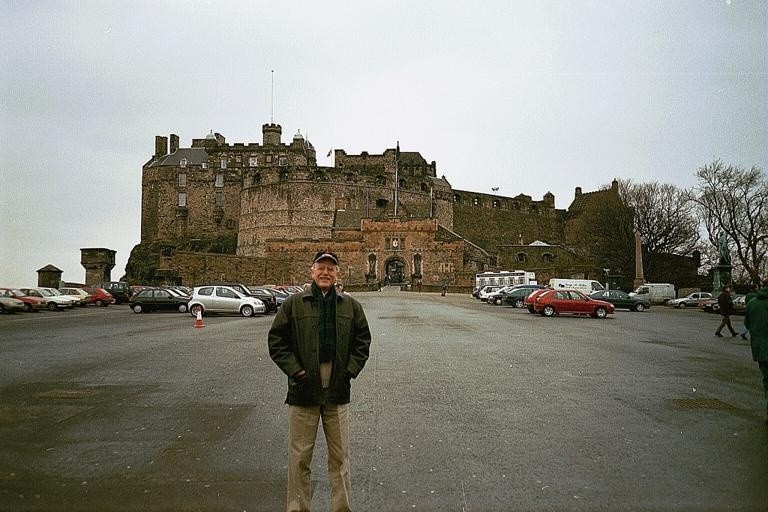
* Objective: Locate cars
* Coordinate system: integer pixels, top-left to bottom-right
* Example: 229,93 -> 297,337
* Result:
697,296 -> 746,315
533,290 -> 614,318
586,290 -> 649,312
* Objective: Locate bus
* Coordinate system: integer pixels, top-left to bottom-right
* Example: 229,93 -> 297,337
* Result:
475,270 -> 528,287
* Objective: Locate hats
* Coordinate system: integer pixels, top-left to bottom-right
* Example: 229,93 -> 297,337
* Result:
313,252 -> 339,266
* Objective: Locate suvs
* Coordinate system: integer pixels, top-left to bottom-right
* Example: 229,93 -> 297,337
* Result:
471,284 -> 546,313
667,292 -> 712,309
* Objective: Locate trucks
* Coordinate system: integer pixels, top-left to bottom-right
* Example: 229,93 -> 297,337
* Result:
628,283 -> 676,306
550,278 -> 604,296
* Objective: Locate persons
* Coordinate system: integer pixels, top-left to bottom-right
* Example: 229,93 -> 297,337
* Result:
267,250 -> 370,512
440,281 -> 447,297
416,280 -> 422,293
713,284 -> 739,338
740,279 -> 768,401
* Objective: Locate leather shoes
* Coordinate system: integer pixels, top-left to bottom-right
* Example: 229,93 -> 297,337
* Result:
715,331 -> 748,340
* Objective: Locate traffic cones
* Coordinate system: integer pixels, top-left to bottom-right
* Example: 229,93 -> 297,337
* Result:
194,306 -> 205,328
